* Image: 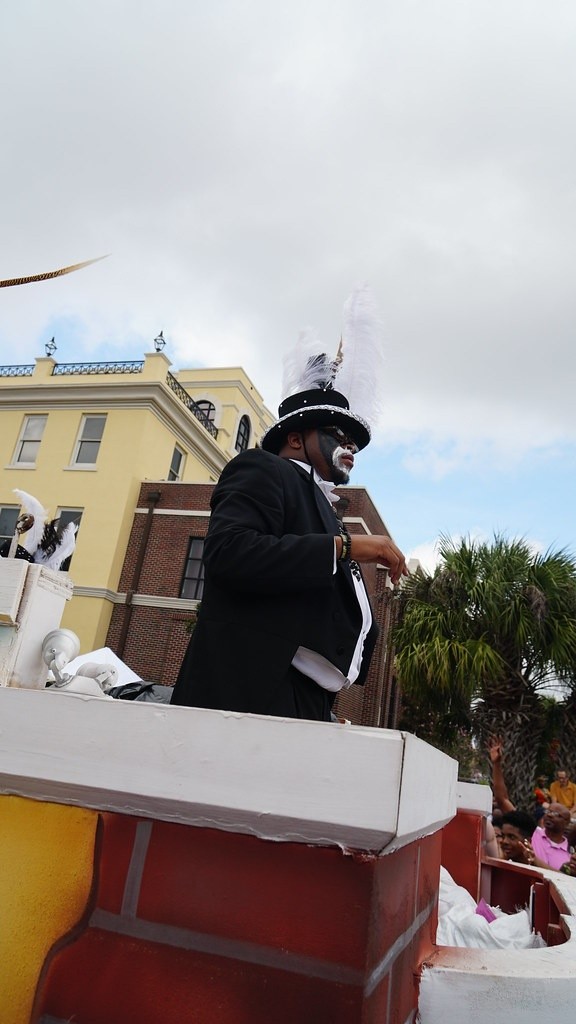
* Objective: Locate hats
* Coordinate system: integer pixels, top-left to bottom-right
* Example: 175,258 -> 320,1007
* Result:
259,334 -> 373,455
492,811 -> 536,837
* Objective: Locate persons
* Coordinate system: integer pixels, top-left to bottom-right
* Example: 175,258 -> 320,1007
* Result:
484,733 -> 576,877
170,334 -> 408,722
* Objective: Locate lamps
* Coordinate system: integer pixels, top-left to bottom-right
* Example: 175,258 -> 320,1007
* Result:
76,661 -> 119,688
150,329 -> 169,352
42,333 -> 60,359
37,627 -> 83,683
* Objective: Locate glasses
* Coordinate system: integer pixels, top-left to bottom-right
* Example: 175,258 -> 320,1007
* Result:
308,421 -> 353,443
494,832 -> 502,838
543,808 -> 570,822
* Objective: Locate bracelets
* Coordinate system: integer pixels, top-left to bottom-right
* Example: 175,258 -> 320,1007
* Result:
339,532 -> 352,562
